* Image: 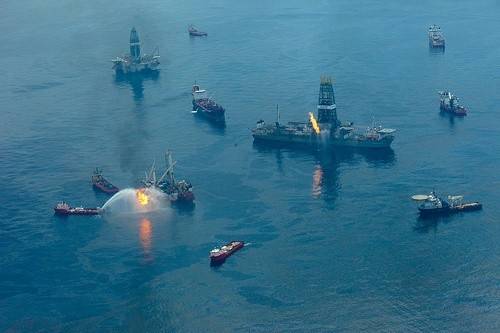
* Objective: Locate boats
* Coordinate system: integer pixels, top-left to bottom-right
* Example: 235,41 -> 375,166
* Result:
411,191 -> 482,214
439,90 -> 467,115
56,202 -> 105,215
91,167 -> 119,193
190,80 -> 227,130
187,25 -> 208,36
427,24 -> 446,52
143,156 -> 196,203
249,77 -> 398,150
112,26 -> 162,75
209,240 -> 245,261
